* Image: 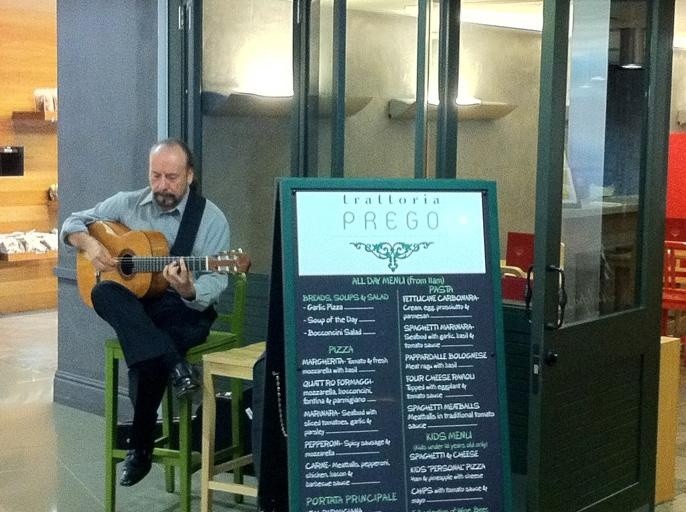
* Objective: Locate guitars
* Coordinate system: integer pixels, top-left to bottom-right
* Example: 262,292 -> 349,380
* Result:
76,222 -> 251,308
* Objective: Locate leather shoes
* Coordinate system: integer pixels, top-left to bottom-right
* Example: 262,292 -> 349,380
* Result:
118,446 -> 153,488
167,362 -> 203,401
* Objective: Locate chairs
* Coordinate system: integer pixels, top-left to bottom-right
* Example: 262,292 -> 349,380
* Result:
103,273 -> 248,512
660,241 -> 686,337
501,265 -> 534,301
202,340 -> 266,512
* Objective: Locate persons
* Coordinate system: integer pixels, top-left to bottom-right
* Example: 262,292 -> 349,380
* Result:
59,140 -> 231,486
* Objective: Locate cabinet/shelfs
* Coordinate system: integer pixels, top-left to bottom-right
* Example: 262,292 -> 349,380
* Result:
655,339 -> 682,505
1,110 -> 58,262
